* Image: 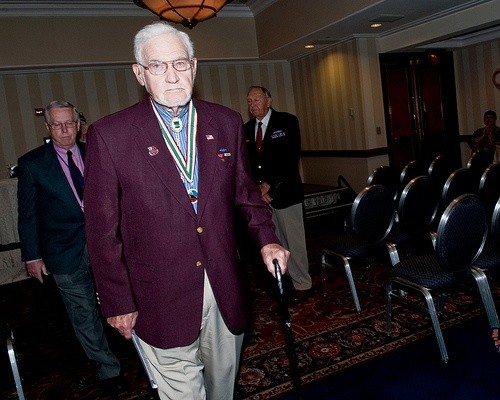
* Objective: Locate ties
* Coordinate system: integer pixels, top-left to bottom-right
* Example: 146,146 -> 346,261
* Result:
66,149 -> 84,202
256,121 -> 263,155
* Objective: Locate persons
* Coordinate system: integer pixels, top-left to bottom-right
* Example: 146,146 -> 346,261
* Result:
17,99 -> 146,400
81,23 -> 291,400
79,113 -> 88,143
470,110 -> 500,171
241,84 -> 313,303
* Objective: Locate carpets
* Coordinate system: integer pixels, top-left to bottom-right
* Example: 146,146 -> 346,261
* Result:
0,258 -> 500,400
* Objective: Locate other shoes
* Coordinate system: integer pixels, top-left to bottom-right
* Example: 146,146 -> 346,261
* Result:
101,373 -> 129,397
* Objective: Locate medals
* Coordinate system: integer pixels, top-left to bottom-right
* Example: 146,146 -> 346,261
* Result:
186,188 -> 198,204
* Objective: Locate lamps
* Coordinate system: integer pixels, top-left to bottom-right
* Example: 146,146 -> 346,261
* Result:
132,0 -> 235,30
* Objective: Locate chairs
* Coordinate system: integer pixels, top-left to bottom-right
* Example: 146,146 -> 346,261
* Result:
320,150 -> 500,369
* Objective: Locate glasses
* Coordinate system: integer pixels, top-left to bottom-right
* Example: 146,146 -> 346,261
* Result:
139,58 -> 194,75
49,120 -> 77,129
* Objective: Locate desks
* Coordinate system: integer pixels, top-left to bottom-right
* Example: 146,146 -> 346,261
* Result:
304,183 -> 355,219
